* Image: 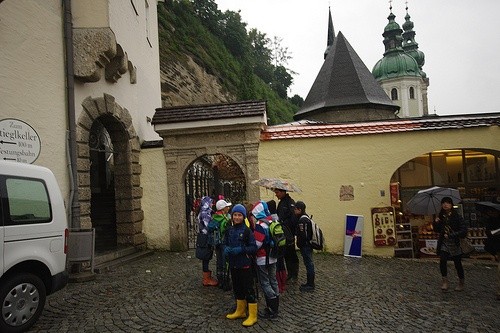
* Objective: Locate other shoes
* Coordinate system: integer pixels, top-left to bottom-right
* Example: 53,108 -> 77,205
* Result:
286,273 -> 298,284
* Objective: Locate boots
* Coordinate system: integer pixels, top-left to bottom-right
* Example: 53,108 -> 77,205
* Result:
242,303 -> 258,326
258,294 -> 280,318
300,276 -> 315,291
455,283 -> 465,291
226,299 -> 246,319
202,270 -> 218,286
276,270 -> 287,293
441,282 -> 449,290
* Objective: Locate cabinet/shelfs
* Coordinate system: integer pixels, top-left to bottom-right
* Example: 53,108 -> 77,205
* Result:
395,223 -> 414,259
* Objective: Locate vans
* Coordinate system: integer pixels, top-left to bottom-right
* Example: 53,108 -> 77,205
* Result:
0,159 -> 69,333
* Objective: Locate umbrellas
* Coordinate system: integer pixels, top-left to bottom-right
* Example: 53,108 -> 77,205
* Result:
252,177 -> 303,203
475,201 -> 500,215
406,186 -> 461,222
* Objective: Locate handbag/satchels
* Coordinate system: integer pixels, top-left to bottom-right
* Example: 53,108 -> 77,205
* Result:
458,237 -> 474,254
196,233 -> 213,260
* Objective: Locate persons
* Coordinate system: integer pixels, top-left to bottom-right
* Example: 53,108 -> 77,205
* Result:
290,200 -> 316,292
195,196 -> 288,293
274,188 -> 299,283
432,197 -> 467,291
484,209 -> 500,262
251,201 -> 281,319
220,204 -> 259,327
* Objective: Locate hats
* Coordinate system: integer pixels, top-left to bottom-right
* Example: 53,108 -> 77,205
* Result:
232,204 -> 246,217
216,199 -> 232,210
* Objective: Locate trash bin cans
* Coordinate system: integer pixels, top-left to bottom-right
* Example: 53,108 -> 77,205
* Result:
68,227 -> 95,263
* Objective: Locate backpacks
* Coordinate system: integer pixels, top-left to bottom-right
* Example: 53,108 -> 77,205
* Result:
299,215 -> 326,250
259,218 -> 295,258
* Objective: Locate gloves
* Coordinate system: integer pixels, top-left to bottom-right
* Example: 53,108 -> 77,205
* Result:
224,246 -> 242,256
